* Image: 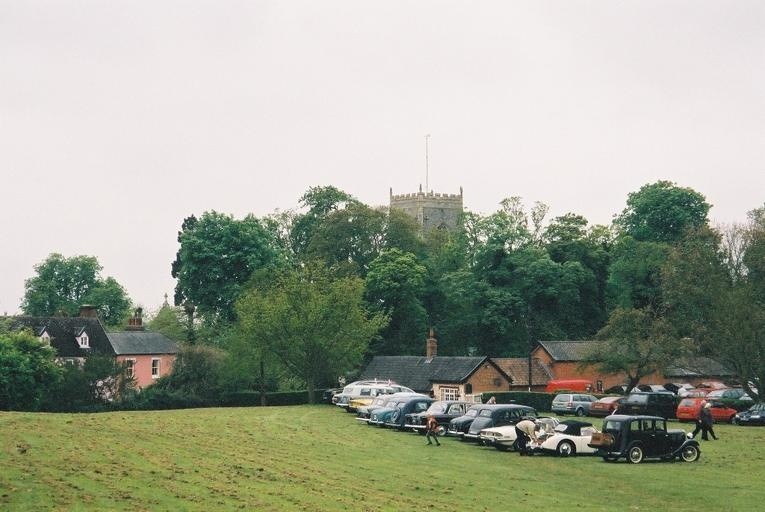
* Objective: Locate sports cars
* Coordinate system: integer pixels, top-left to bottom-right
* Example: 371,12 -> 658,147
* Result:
537,419 -> 600,457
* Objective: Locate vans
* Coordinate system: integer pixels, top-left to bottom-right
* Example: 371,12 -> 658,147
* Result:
619,391 -> 678,419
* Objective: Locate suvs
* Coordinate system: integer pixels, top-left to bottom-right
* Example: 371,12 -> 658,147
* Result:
551,394 -> 600,417
332,379 -> 434,428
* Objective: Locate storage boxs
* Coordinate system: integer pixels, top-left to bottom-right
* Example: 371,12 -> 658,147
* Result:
591,433 -> 611,446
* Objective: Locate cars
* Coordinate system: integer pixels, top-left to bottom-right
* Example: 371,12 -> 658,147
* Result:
603,380 -> 730,399
464,403 -> 539,440
404,400 -> 472,436
675,397 -> 737,423
589,396 -> 628,417
587,414 -> 700,464
735,402 -> 765,426
478,415 -> 560,447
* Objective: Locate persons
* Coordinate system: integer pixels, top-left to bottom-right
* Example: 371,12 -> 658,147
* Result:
487,397 -> 497,405
701,402 -> 720,441
425,415 -> 441,446
515,420 -> 544,457
689,400 -> 709,440
